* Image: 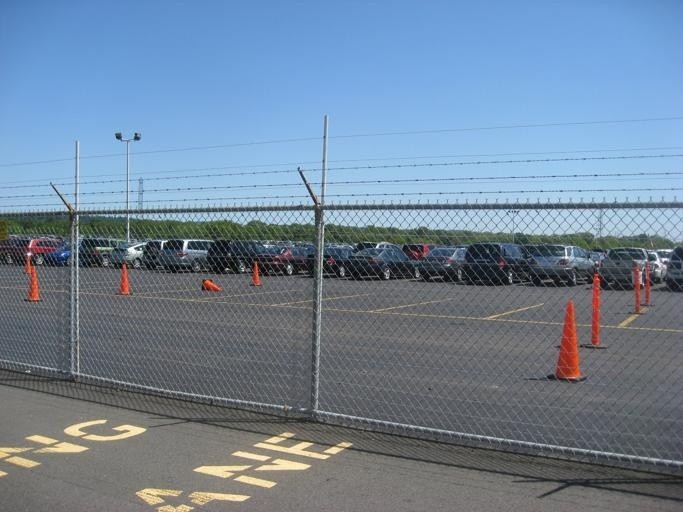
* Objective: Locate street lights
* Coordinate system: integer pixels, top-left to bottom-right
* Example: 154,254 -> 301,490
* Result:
507,211 -> 519,243
115,131 -> 141,242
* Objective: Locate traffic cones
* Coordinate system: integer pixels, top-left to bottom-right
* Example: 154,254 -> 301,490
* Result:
23,266 -> 43,303
22,256 -> 32,275
114,263 -> 134,296
546,299 -> 585,383
201,278 -> 222,293
